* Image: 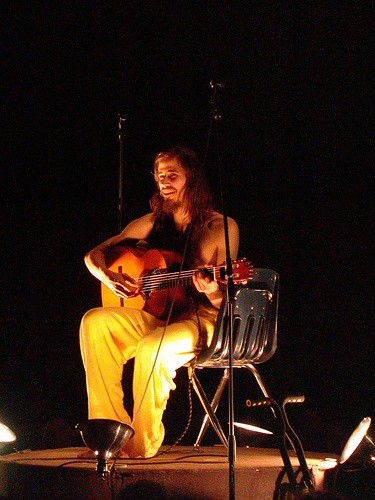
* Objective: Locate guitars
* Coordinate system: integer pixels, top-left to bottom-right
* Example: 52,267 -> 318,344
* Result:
99,244 -> 254,317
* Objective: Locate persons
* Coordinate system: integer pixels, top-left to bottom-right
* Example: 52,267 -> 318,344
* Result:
79,144 -> 241,461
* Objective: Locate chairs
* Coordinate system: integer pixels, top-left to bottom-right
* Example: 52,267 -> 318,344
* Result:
188,268 -> 298,451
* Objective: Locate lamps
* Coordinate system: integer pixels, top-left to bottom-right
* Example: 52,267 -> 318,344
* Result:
0,422 -> 18,455
75,418 -> 136,473
340,417 -> 375,470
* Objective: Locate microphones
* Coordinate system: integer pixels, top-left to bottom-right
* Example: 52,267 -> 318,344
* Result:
210,77 -> 224,120
